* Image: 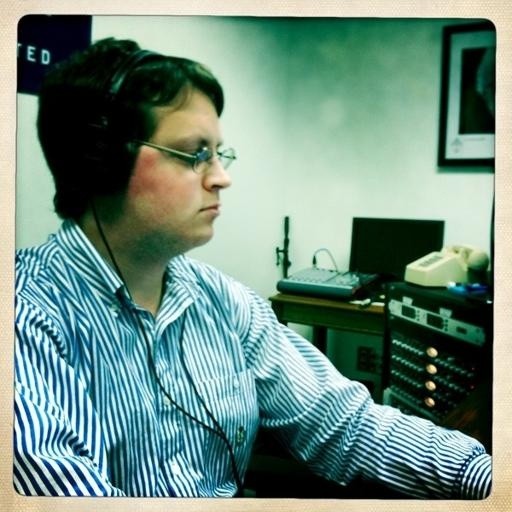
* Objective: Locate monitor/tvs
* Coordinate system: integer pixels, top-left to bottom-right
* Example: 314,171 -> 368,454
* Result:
348,218 -> 445,290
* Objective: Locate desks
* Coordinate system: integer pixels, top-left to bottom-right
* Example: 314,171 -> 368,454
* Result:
268,293 -> 385,358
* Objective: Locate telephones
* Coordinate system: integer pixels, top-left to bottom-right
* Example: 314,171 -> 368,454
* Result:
405,243 -> 489,287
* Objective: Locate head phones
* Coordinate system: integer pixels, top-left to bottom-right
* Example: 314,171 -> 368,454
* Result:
63,50 -> 157,198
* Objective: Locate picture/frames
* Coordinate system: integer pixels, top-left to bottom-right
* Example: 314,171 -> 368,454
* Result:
437,23 -> 495,167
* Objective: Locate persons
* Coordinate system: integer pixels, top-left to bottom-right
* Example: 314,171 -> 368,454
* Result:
14,33 -> 491,497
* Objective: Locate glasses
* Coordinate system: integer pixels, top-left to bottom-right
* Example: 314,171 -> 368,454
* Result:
141,139 -> 236,175
